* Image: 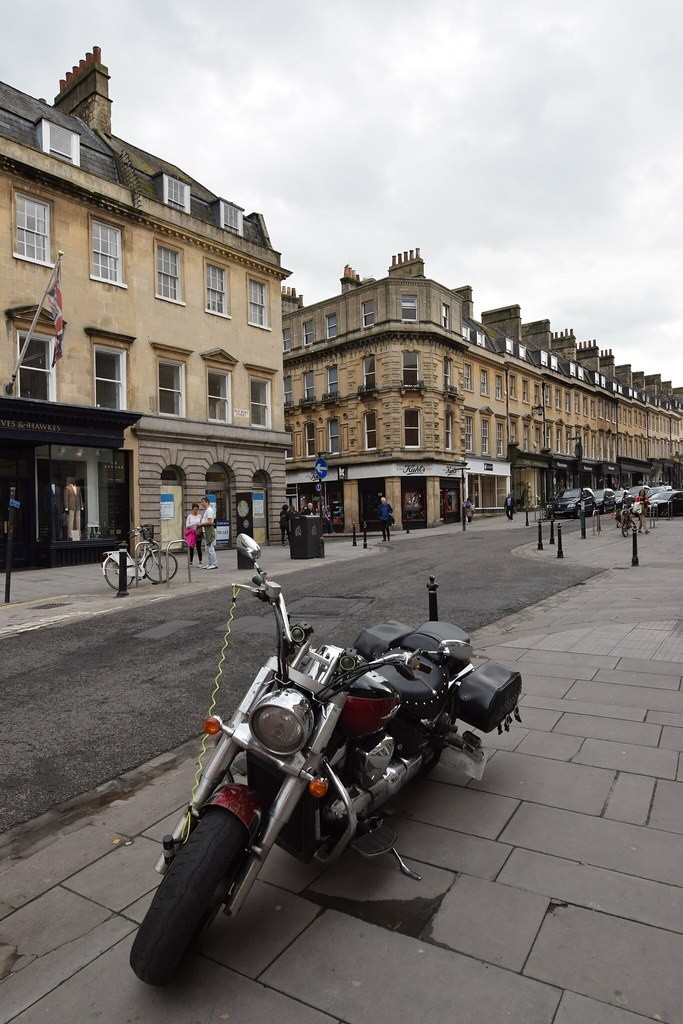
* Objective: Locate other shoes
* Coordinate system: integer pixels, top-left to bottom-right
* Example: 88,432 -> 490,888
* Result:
197,562 -> 203,568
388,538 -> 390,541
645,531 -> 650,534
382,539 -> 386,541
207,565 -> 218,570
202,564 -> 211,569
190,562 -> 192,567
639,531 -> 643,533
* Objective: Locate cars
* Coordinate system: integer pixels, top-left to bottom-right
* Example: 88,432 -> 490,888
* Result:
640,491 -> 683,517
592,487 -> 617,515
615,489 -> 634,509
628,485 -> 676,505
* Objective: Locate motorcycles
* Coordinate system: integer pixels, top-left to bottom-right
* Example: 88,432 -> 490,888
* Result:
126,532 -> 524,987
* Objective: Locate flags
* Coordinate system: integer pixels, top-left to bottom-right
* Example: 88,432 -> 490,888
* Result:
47,262 -> 65,367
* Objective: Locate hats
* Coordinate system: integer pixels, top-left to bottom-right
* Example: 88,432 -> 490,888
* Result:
307,503 -> 312,506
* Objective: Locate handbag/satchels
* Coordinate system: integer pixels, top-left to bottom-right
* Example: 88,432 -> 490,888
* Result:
387,515 -> 394,526
196,526 -> 204,542
354,619 -> 416,663
452,659 -> 522,733
470,505 -> 475,513
468,512 -> 473,518
213,519 -> 217,528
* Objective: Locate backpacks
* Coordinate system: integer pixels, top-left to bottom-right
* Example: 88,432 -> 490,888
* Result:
280,515 -> 287,528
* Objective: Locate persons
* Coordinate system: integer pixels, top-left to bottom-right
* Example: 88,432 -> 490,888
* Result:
186,503 -> 203,568
620,489 -> 651,534
196,497 -> 219,570
279,502 -> 336,547
504,493 -> 515,522
378,497 -> 393,541
464,498 -> 476,524
44,479 -> 85,541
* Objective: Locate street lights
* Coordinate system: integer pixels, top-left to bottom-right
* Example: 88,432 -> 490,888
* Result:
454,466 -> 473,532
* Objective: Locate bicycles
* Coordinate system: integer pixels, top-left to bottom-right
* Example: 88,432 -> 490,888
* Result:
621,497 -> 638,537
101,523 -> 180,591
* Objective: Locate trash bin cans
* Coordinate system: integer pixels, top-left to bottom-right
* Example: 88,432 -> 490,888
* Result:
289,514 -> 322,559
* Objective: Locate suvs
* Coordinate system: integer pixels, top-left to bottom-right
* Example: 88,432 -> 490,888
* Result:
546,486 -> 597,520
